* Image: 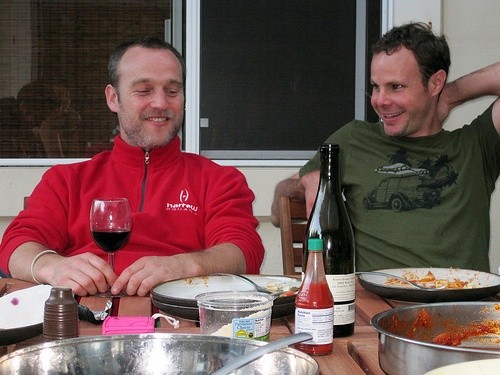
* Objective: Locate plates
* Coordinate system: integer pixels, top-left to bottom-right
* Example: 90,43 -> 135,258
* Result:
359,267 -> 500,302
150,272 -> 300,321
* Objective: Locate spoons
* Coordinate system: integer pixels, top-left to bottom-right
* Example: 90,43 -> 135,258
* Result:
354,270 -> 448,291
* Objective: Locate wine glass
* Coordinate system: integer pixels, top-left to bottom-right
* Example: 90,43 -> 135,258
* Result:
90,196 -> 132,299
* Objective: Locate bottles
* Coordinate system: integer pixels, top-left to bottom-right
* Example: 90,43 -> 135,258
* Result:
294,238 -> 334,356
301,141 -> 357,337
40,286 -> 79,345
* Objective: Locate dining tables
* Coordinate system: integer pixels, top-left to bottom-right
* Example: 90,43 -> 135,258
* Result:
0,275 -> 500,375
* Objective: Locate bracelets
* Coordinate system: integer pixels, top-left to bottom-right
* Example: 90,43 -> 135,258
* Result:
31,249 -> 56,285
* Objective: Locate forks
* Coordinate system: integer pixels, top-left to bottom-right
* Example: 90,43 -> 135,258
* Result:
210,271 -> 285,295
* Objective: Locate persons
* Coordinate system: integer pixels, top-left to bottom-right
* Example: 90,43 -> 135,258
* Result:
16,64 -> 66,157
271,23 -> 500,275
0,37 -> 264,297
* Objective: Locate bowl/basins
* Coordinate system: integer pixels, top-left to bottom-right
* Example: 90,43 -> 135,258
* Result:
0,333 -> 322,375
194,290 -> 277,342
369,300 -> 500,375
